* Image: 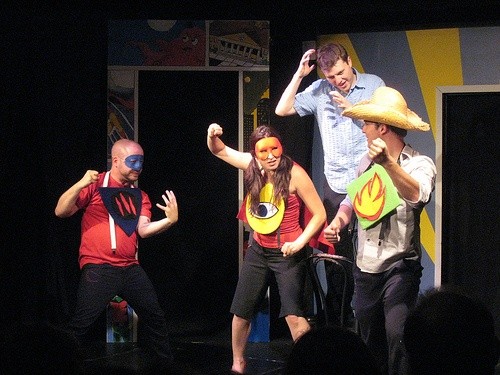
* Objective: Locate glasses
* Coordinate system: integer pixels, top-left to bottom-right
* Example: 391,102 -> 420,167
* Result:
359,121 -> 379,129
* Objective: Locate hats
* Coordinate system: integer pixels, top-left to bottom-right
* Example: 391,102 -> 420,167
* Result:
341,86 -> 430,132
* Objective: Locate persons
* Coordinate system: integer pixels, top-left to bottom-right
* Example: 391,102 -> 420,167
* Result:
55,139 -> 179,375
108,295 -> 130,343
0,289 -> 500,375
208,123 -> 327,374
275,41 -> 387,325
324,85 -> 437,375
